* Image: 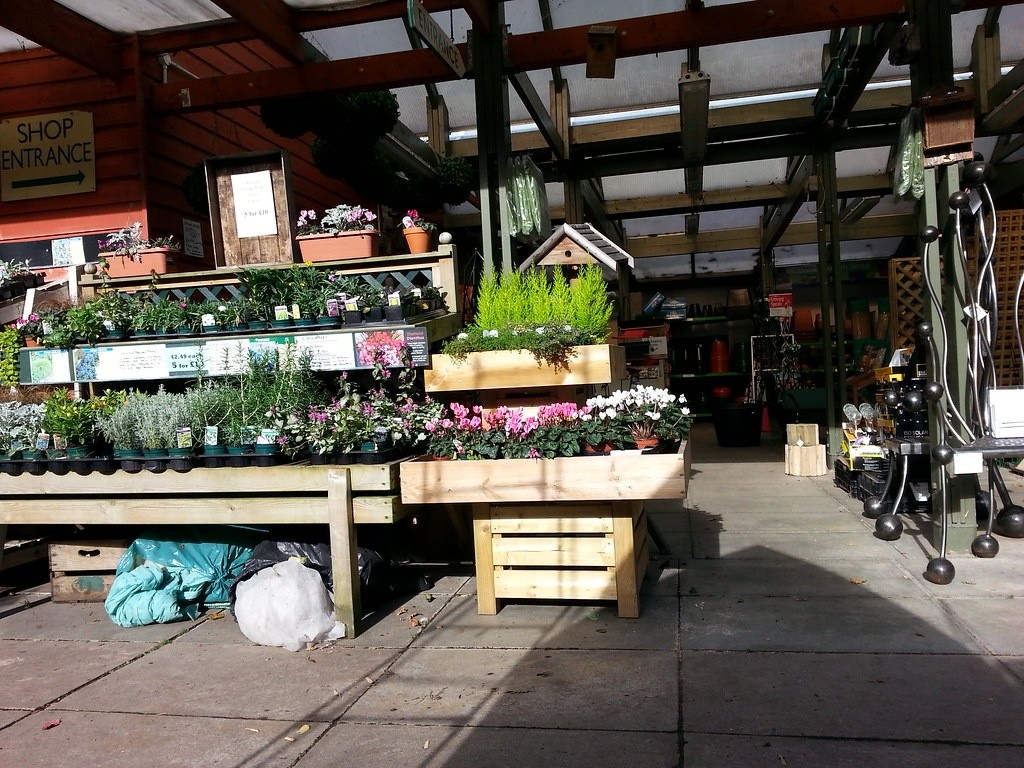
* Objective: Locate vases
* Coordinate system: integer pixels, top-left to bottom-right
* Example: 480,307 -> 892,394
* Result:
173,324 -> 200,333
66,445 -> 92,476
633,436 -> 659,449
335,444 -> 355,465
433,455 -> 452,461
295,229 -> 382,262
98,248 -> 180,279
46,449 -> 68,475
317,315 -> 344,325
620,288 -> 877,448
659,437 -> 682,453
292,318 -> 317,326
33,272 -> 47,287
403,227 -> 432,254
25,335 -> 44,347
358,441 -> 385,464
581,439 -> 616,456
307,443 -> 325,465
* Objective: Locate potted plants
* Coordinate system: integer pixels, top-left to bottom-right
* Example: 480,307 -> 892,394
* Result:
400,292 -> 415,317
195,300 -> 223,332
221,298 -> 248,330
267,268 -> 294,328
4,258 -> 25,296
152,298 -> 176,334
133,384 -> 170,472
410,296 -> 420,316
188,387 -> 225,467
418,287 -> 436,312
432,286 -> 446,309
232,266 -> 272,329
364,285 -> 387,322
384,287 -> 406,321
23,258 -> 35,288
8,403 -> 47,475
221,386 -> 252,467
91,257 -> 131,338
0,401 -> 22,476
340,275 -> 367,324
94,387 -> 142,473
0,260 -> 11,301
247,383 -> 280,466
166,387 -> 197,471
127,294 -> 154,335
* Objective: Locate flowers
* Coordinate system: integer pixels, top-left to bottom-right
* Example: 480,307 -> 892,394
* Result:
97,221 -> 182,264
450,395 -> 625,460
264,340 -> 449,461
425,417 -> 455,459
11,311 -> 42,337
172,296 -> 199,327
397,209 -> 438,232
70,389 -> 126,445
289,260 -> 316,318
308,267 -> 346,318
296,204 -> 377,235
39,386 -> 72,447
612,385 -> 697,435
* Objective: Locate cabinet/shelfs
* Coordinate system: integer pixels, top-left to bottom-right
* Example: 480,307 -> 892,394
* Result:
778,339 -> 891,374
634,314 -> 752,418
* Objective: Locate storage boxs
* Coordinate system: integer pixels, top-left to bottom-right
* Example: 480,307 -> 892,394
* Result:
202,148 -> 302,270
857,470 -> 931,514
833,460 -> 859,499
46,539 -> 129,603
